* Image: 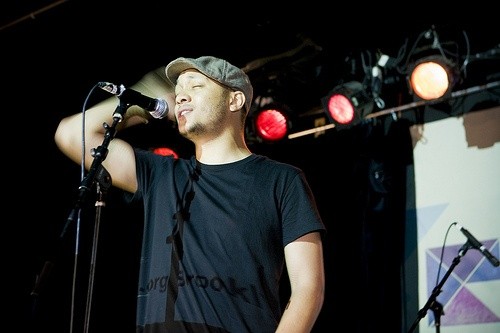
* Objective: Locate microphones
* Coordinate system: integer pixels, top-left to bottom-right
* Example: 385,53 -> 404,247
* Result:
454,224 -> 499,266
99,81 -> 169,120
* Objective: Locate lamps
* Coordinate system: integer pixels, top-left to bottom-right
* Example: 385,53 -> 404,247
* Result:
409,40 -> 460,105
251,97 -> 296,142
320,80 -> 382,128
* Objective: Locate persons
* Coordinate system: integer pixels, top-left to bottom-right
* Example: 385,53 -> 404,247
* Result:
54,56 -> 327,333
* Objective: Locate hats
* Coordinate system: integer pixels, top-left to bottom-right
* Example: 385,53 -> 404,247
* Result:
165,55 -> 254,112
